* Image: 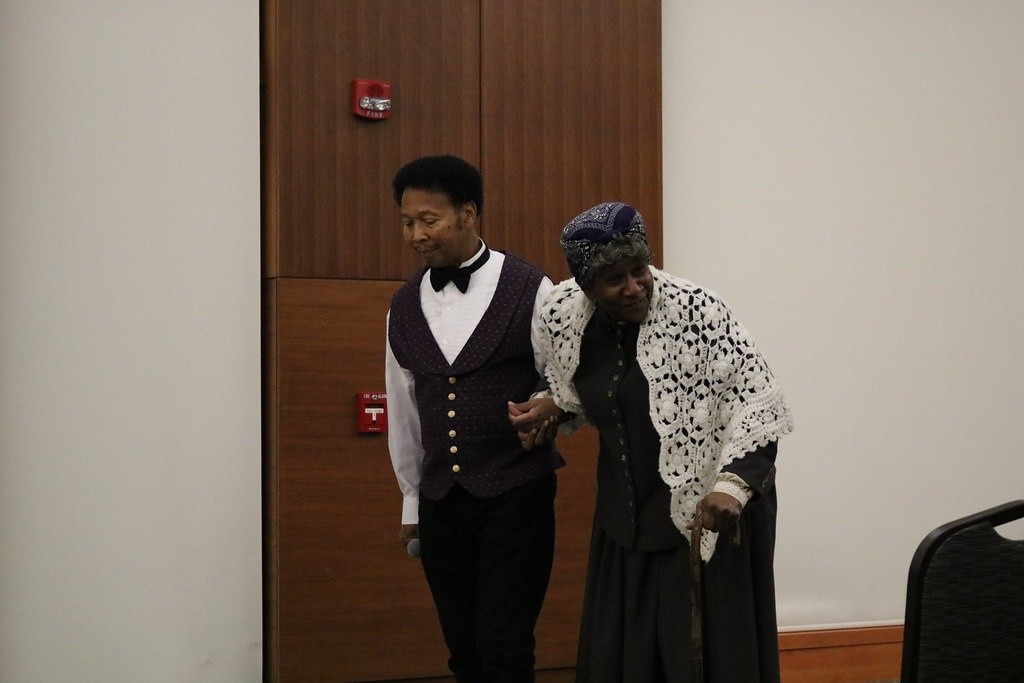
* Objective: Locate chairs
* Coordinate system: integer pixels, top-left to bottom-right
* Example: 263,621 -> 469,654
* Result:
899,498 -> 1024,683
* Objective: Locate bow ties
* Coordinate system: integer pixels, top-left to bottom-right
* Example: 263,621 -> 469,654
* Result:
427,246 -> 491,294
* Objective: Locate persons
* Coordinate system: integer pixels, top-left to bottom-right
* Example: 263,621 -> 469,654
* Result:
508,202 -> 793,683
385,155 -> 566,683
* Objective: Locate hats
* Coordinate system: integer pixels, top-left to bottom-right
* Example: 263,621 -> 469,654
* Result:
560,202 -> 650,290
